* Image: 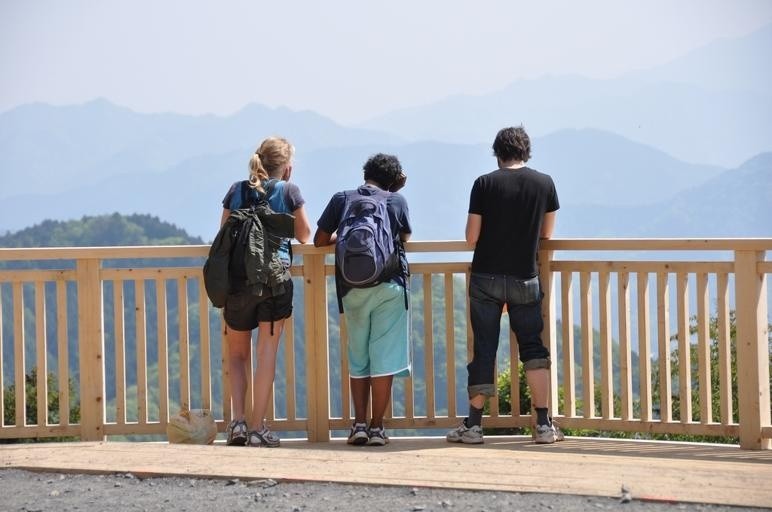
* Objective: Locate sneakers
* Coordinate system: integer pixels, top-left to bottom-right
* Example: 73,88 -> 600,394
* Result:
224,419 -> 279,449
347,421 -> 388,447
535,417 -> 565,444
447,417 -> 484,445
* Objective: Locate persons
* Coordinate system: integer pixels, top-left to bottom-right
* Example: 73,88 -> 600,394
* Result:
219,137 -> 311,448
446,127 -> 566,444
313,154 -> 412,446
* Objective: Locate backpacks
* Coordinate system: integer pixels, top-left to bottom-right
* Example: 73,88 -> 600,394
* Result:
335,188 -> 410,313
226,177 -> 280,288
